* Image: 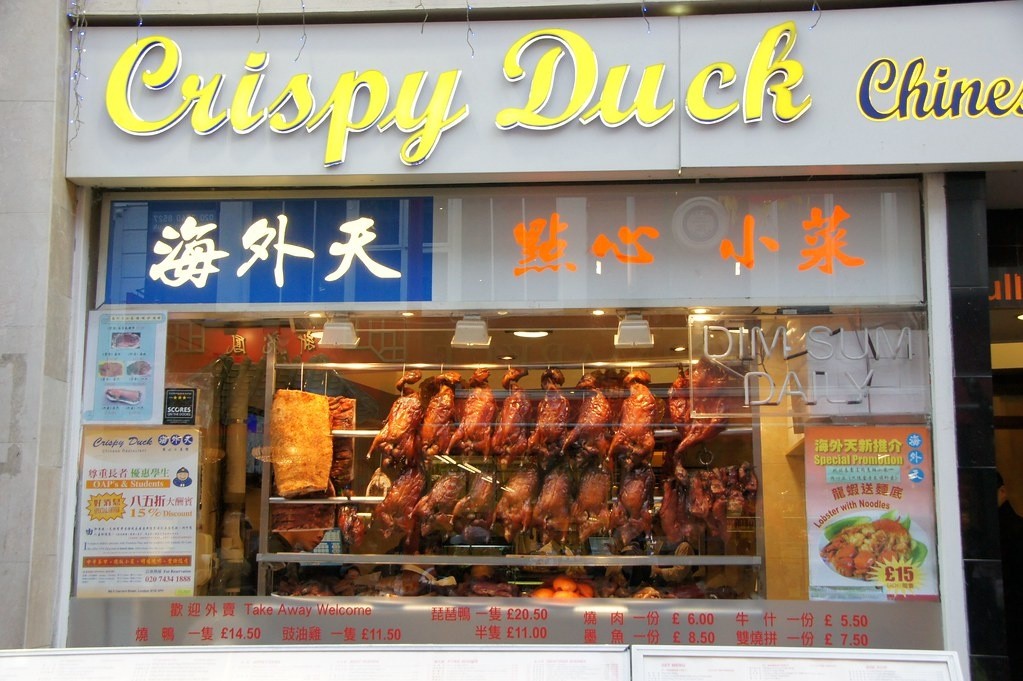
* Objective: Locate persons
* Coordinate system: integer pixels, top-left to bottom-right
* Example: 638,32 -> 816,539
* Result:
377,535 -> 441,595
540,541 -> 575,556
462,527 -> 488,543
997,473 -> 1022,681
251,479 -> 337,592
601,534 -> 696,596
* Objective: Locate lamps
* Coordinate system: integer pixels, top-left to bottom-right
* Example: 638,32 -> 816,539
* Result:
451,314 -> 492,349
318,312 -> 360,350
613,308 -> 654,349
